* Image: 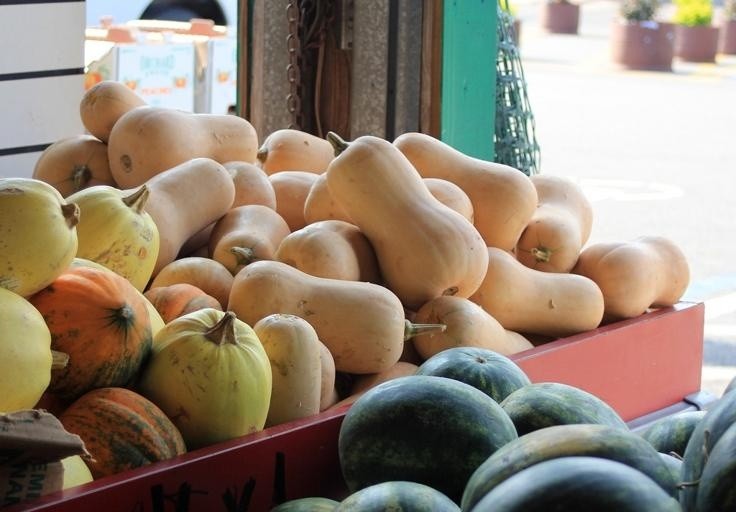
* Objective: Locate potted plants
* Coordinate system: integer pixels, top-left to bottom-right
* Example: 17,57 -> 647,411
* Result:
611,0 -> 676,69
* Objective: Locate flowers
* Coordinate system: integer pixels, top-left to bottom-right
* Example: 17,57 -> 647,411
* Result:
673,0 -> 715,26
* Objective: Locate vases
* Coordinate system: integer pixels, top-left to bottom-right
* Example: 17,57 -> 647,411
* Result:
547,5 -> 579,33
720,21 -> 736,54
674,24 -> 718,62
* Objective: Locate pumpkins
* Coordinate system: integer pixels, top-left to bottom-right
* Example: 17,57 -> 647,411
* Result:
0,79 -> 690,490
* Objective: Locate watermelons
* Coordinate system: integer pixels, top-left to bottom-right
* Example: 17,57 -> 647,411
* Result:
267,346 -> 736,512
